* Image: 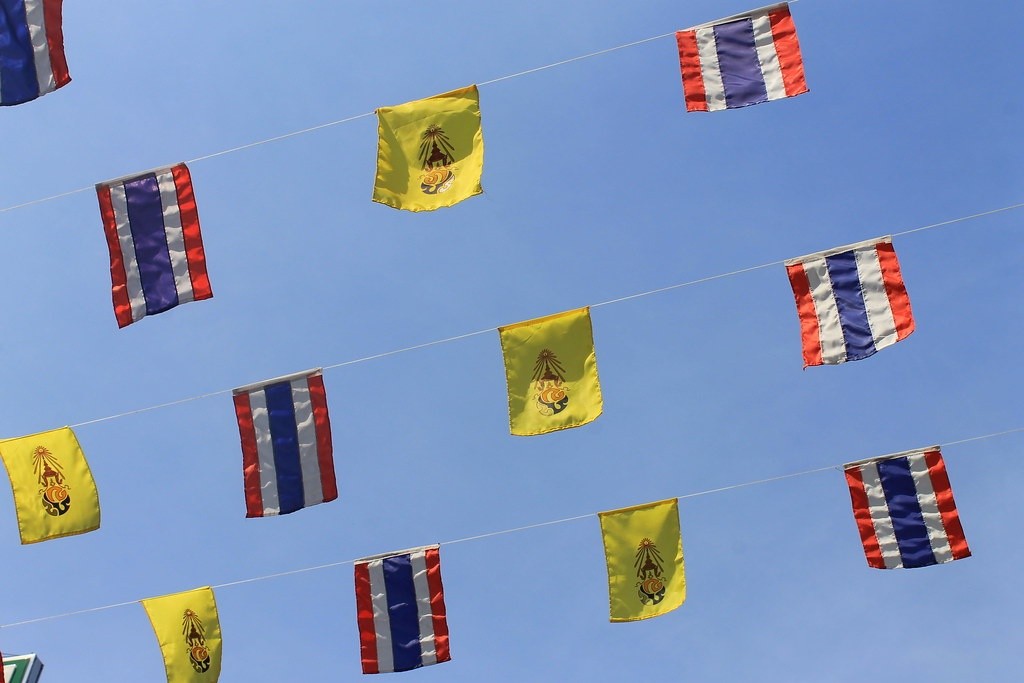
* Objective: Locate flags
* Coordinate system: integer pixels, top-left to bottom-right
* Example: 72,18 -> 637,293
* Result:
843,446 -> 972,568
371,83 -> 484,213
1,426 -> 100,544
0,0 -> 73,107
598,498 -> 686,622
783,233 -> 915,369
95,162 -> 213,328
354,544 -> 451,673
496,306 -> 602,435
676,0 -> 810,111
233,366 -> 339,518
141,586 -> 222,683
